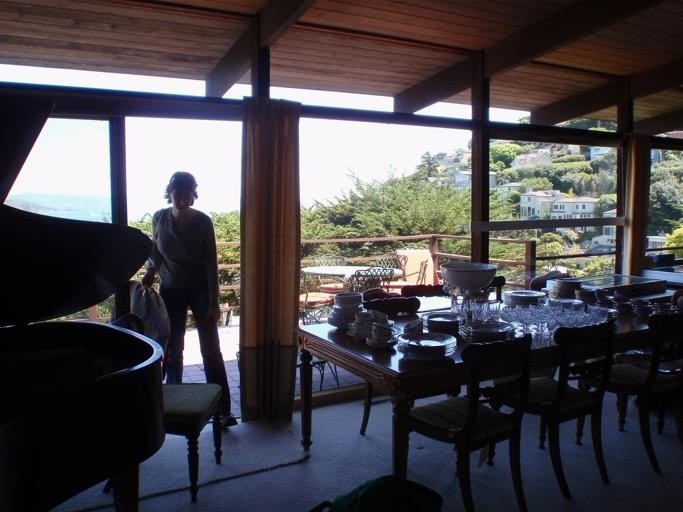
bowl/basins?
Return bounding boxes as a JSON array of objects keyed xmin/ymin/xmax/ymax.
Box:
[
  {"xmin": 440, "ymin": 261, "xmax": 498, "ymax": 295},
  {"xmin": 328, "ymin": 292, "xmax": 396, "ymax": 348},
  {"xmin": 572, "ymin": 288, "xmax": 681, "ymax": 320}
]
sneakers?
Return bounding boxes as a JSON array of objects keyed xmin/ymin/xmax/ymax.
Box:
[{"xmin": 220, "ymin": 415, "xmax": 238, "ymax": 427}]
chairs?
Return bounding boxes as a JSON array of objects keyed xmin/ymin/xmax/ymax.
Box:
[
  {"xmin": 359, "ymin": 271, "xmax": 682, "ymax": 510},
  {"xmin": 102, "ymin": 313, "xmax": 224, "ymax": 502}
]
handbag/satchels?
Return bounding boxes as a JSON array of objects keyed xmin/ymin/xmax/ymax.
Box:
[{"xmin": 148, "ymin": 240, "xmax": 161, "ymax": 272}]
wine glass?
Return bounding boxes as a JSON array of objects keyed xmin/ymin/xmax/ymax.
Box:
[{"xmin": 459, "ymin": 297, "xmax": 609, "ymax": 348}]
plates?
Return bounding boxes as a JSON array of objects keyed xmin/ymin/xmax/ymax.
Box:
[
  {"xmin": 506, "ymin": 277, "xmax": 582, "ymax": 308},
  {"xmin": 397, "ymin": 312, "xmax": 516, "ymax": 362}
]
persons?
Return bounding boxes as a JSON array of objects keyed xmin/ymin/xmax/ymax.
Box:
[{"xmin": 142, "ymin": 171, "xmax": 239, "ymax": 430}]
